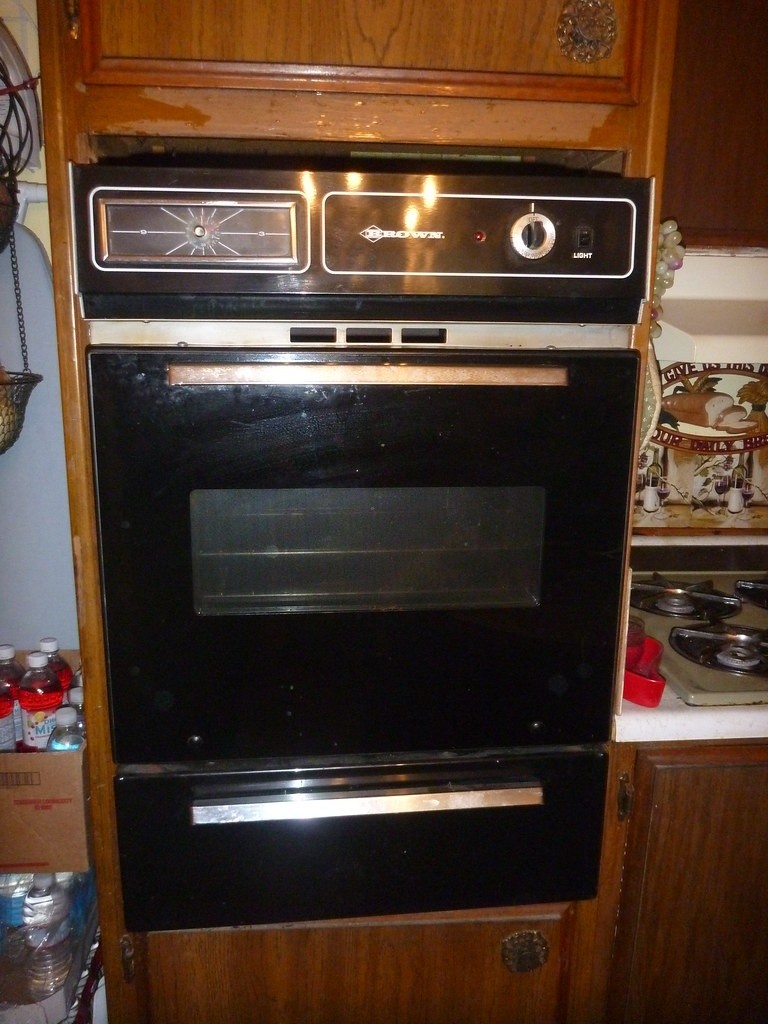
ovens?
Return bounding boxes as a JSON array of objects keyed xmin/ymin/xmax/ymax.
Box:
[{"xmin": 68, "ymin": 160, "xmax": 657, "ymax": 768}]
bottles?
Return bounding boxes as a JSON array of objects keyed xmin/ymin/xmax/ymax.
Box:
[
  {"xmin": 39, "ymin": 637, "xmax": 74, "ymax": 708},
  {"xmin": 66, "ymin": 686, "xmax": 84, "ymax": 734},
  {"xmin": 0, "ymin": 680, "xmax": 17, "ymax": 753},
  {"xmin": 72, "ymin": 660, "xmax": 84, "ymax": 687},
  {"xmin": 22, "ymin": 872, "xmax": 72, "ymax": 1002},
  {"xmin": 18, "ymin": 651, "xmax": 64, "ymax": 751},
  {"xmin": 727, "ymin": 452, "xmax": 748, "ymax": 513},
  {"xmin": 642, "ymin": 447, "xmax": 663, "ymax": 513},
  {"xmin": 0, "ymin": 644, "xmax": 27, "ymax": 753},
  {"xmin": 46, "ymin": 706, "xmax": 85, "ymax": 751}
]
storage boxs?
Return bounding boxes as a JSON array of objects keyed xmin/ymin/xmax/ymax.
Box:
[{"xmin": 0, "ymin": 648, "xmax": 91, "ymax": 874}]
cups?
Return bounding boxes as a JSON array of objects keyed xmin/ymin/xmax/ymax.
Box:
[
  {"xmin": 625, "ymin": 615, "xmax": 645, "ymax": 672},
  {"xmin": 727, "ymin": 486, "xmax": 745, "ymax": 514},
  {"xmin": 642, "ymin": 484, "xmax": 661, "ymax": 513}
]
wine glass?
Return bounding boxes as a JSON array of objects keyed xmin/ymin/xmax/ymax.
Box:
[
  {"xmin": 738, "ymin": 479, "xmax": 755, "ymax": 520},
  {"xmin": 653, "ymin": 477, "xmax": 671, "ymax": 519},
  {"xmin": 714, "ymin": 480, "xmax": 728, "ymax": 514},
  {"xmin": 633, "ymin": 474, "xmax": 647, "ymax": 521}
]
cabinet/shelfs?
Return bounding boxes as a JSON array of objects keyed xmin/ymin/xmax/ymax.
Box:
[{"xmin": 36, "ymin": 0, "xmax": 768, "ymax": 1024}]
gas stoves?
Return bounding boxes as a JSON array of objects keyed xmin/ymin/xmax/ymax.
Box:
[{"xmin": 631, "ymin": 570, "xmax": 768, "ymax": 705}]
